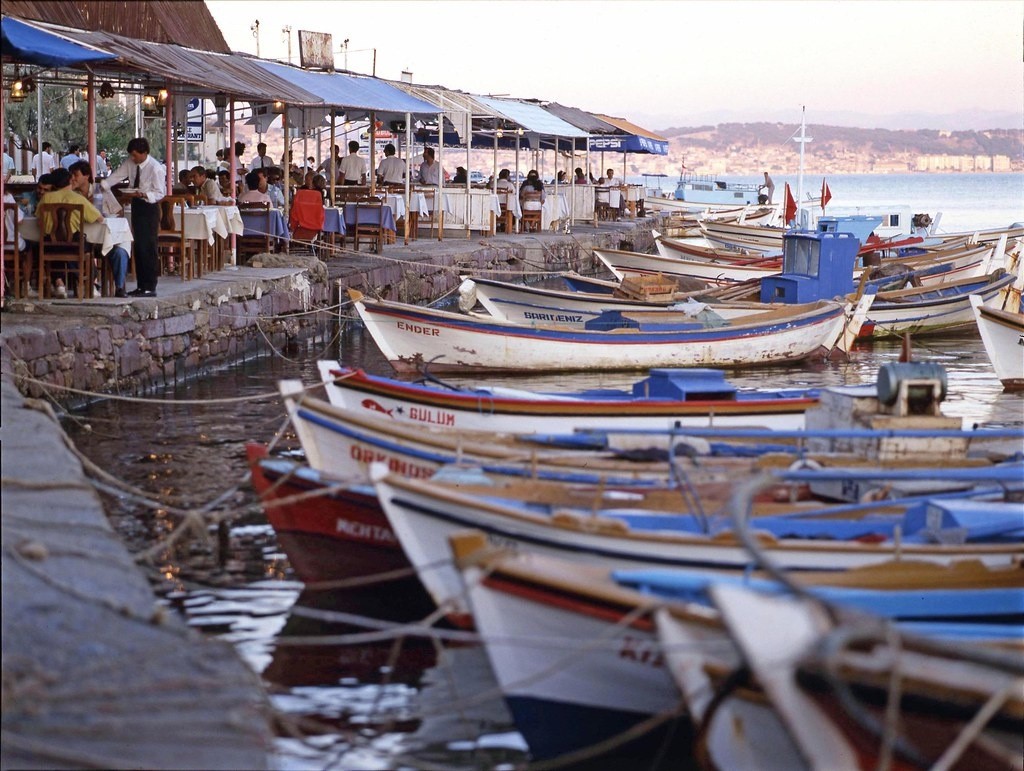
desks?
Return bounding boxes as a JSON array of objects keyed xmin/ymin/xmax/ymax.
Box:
[
  {"xmin": 621, "ymin": 187, "xmax": 646, "ymax": 217},
  {"xmin": 424, "ymin": 193, "xmax": 452, "ymax": 239},
  {"xmin": 500, "ymin": 193, "xmax": 523, "ymax": 234},
  {"xmin": 16, "ymin": 216, "xmax": 134, "ymax": 300},
  {"xmin": 124, "ymin": 206, "xmax": 228, "ymax": 282},
  {"xmin": 364, "ymin": 195, "xmax": 406, "ymax": 225},
  {"xmin": 540, "ymin": 194, "xmax": 571, "ymax": 234},
  {"xmin": 597, "ymin": 188, "xmax": 621, "ymax": 222},
  {"xmin": 241, "ymin": 208, "xmax": 290, "ymax": 246},
  {"xmin": 322, "ymin": 208, "xmax": 346, "ymax": 256},
  {"xmin": 195, "ymin": 205, "xmax": 244, "ymax": 273},
  {"xmin": 335, "ymin": 203, "xmax": 397, "ymax": 253},
  {"xmin": 400, "ymin": 193, "xmax": 429, "ymax": 241},
  {"xmin": 416, "ymin": 187, "xmax": 501, "ymax": 237}
]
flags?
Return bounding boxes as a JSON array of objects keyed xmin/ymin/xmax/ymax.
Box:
[
  {"xmin": 785, "ymin": 185, "xmax": 798, "ymax": 226},
  {"xmin": 821, "ymin": 181, "xmax": 832, "ymax": 209}
]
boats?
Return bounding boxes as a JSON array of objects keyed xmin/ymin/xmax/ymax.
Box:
[{"xmin": 244, "ymin": 104, "xmax": 1024, "ymax": 771}]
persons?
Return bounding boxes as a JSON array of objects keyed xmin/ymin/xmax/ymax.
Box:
[
  {"xmin": 105, "ymin": 137, "xmax": 166, "ymax": 297},
  {"xmin": 60, "ymin": 144, "xmax": 81, "ymax": 170},
  {"xmin": 216, "ymin": 141, "xmax": 284, "ymax": 260},
  {"xmin": 316, "ymin": 145, "xmax": 342, "ymax": 183},
  {"xmin": 85, "ymin": 144, "xmax": 111, "ymax": 176},
  {"xmin": 550, "ymin": 167, "xmax": 626, "ymax": 221},
  {"xmin": 418, "ymin": 147, "xmax": 444, "ymax": 185},
  {"xmin": 377, "ymin": 144, "xmax": 405, "ymax": 186},
  {"xmin": 453, "ymin": 167, "xmax": 467, "ymax": 183},
  {"xmin": 488, "ymin": 168, "xmax": 546, "ymax": 232},
  {"xmin": 32, "ymin": 142, "xmax": 54, "ymax": 182},
  {"xmin": 173, "ymin": 166, "xmax": 230, "ymax": 206},
  {"xmin": 338, "ymin": 140, "xmax": 367, "ymax": 185},
  {"xmin": 3, "ymin": 158, "xmax": 128, "ymax": 299},
  {"xmin": 3, "ymin": 144, "xmax": 16, "ymax": 182},
  {"xmin": 281, "ymin": 150, "xmax": 332, "ymax": 206},
  {"xmin": 760, "ymin": 172, "xmax": 775, "ymax": 205}
]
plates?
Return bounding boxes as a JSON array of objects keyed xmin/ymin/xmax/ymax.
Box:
[{"xmin": 117, "ymin": 188, "xmax": 139, "ymax": 193}]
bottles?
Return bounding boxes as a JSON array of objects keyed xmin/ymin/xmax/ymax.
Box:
[
  {"xmin": 194, "ymin": 188, "xmax": 201, "ymax": 206},
  {"xmin": 93, "ymin": 184, "xmax": 103, "ymax": 213}
]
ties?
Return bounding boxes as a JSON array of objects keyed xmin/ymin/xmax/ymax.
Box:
[
  {"xmin": 261, "ymin": 157, "xmax": 264, "ymax": 168},
  {"xmin": 134, "ymin": 166, "xmax": 140, "ymax": 189}
]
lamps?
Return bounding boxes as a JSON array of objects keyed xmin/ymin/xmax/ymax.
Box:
[{"xmin": 10, "ymin": 65, "xmax": 525, "ymax": 138}]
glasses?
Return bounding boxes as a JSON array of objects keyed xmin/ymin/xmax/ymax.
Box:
[{"xmin": 272, "ymin": 178, "xmax": 279, "ymax": 181}]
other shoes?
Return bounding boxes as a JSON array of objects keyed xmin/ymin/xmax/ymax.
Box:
[
  {"xmin": 617, "ymin": 217, "xmax": 621, "ymax": 222},
  {"xmin": 21, "ymin": 288, "xmax": 38, "ymax": 298},
  {"xmin": 528, "ymin": 224, "xmax": 536, "ymax": 233},
  {"xmin": 625, "ymin": 208, "xmax": 633, "ymax": 216},
  {"xmin": 500, "ymin": 222, "xmax": 505, "ymax": 232},
  {"xmin": 55, "ymin": 286, "xmax": 68, "ymax": 299},
  {"xmin": 93, "ymin": 286, "xmax": 101, "ymax": 297}
]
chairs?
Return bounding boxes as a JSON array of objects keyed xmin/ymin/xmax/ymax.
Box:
[{"xmin": 1, "ymin": 185, "xmax": 645, "ymax": 298}]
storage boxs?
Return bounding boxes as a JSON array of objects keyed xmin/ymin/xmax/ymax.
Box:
[{"xmin": 613, "ymin": 272, "xmax": 679, "ymax": 302}]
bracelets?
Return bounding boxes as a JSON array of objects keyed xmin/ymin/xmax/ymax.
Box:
[{"xmin": 142, "ymin": 193, "xmax": 143, "ymax": 198}]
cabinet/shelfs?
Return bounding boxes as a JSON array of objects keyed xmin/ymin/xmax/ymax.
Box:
[{"xmin": 806, "ymin": 387, "xmax": 968, "ymax": 504}]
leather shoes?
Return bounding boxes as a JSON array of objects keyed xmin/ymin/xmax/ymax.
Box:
[
  {"xmin": 133, "ymin": 290, "xmax": 156, "ymax": 297},
  {"xmin": 116, "ymin": 289, "xmax": 128, "ymax": 298},
  {"xmin": 128, "ymin": 288, "xmax": 141, "ymax": 295}
]
